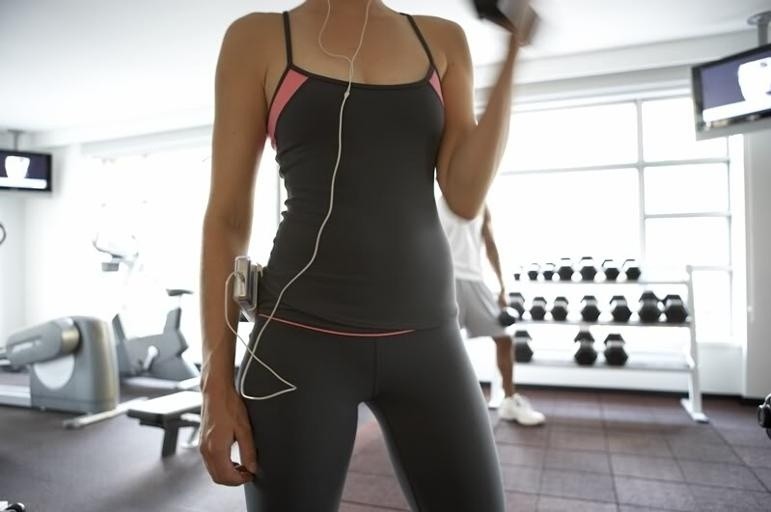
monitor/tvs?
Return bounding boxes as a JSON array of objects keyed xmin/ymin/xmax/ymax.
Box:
[
  {"xmin": 0, "ymin": 148, "xmax": 51, "ymax": 192},
  {"xmin": 689, "ymin": 42, "xmax": 770, "ymax": 140}
]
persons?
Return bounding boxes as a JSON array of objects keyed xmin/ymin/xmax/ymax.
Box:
[
  {"xmin": 428, "ymin": 173, "xmax": 547, "ymax": 425},
  {"xmin": 199, "ymin": 0, "xmax": 535, "ymax": 510}
]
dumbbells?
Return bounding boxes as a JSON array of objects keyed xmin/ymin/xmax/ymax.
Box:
[
  {"xmin": 510, "ymin": 330, "xmax": 533, "ymax": 364},
  {"xmin": 572, "ymin": 330, "xmax": 596, "ymax": 369},
  {"xmin": 496, "ymin": 254, "xmax": 640, "ymax": 281},
  {"xmin": 601, "ymin": 333, "xmax": 629, "ymax": 369},
  {"xmin": 502, "ymin": 292, "xmax": 690, "ymax": 326}
]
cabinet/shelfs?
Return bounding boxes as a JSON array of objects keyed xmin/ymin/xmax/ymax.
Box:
[{"xmin": 487, "ymin": 264, "xmax": 709, "ymax": 424}]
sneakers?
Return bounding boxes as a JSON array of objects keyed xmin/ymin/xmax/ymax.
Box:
[{"xmin": 499, "ymin": 392, "xmax": 546, "ymax": 427}]
